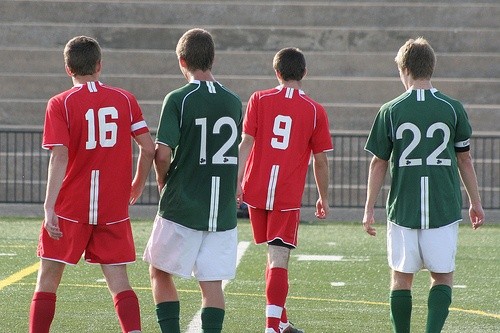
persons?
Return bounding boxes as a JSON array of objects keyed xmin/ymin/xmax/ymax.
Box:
[
  {"xmin": 30, "ymin": 35, "xmax": 156, "ymax": 332},
  {"xmin": 362, "ymin": 35, "xmax": 485, "ymax": 333},
  {"xmin": 142, "ymin": 29, "xmax": 243, "ymax": 333},
  {"xmin": 236, "ymin": 46, "xmax": 332, "ymax": 333}
]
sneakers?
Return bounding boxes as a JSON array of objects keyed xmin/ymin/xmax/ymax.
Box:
[{"xmin": 279, "ymin": 321, "xmax": 304, "ymax": 333}]
